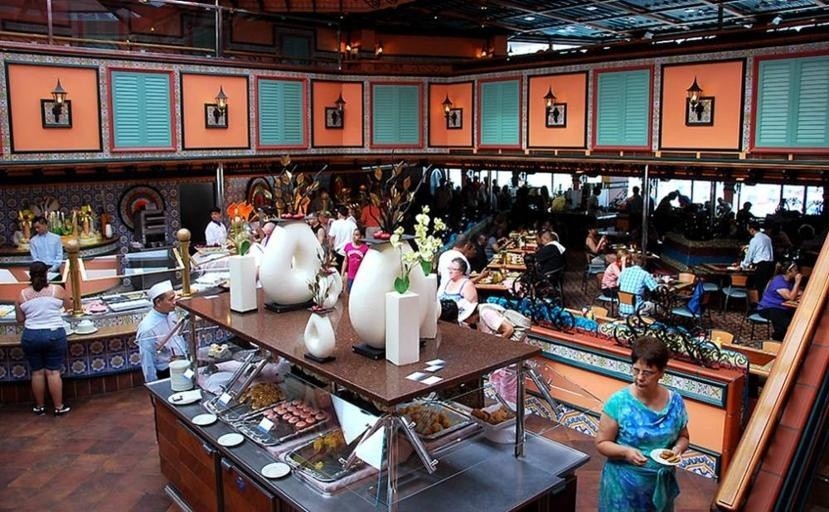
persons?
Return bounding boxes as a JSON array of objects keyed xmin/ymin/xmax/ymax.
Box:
[
  {"xmin": 594, "ymin": 336, "xmax": 690, "ymax": 512},
  {"xmin": 204, "ymin": 207, "xmax": 276, "ymax": 256},
  {"xmin": 136, "ymin": 278, "xmax": 188, "ymax": 384},
  {"xmin": 15, "ymin": 260, "xmax": 70, "ymax": 415},
  {"xmin": 306, "ymin": 198, "xmax": 381, "ymax": 289},
  {"xmin": 28, "ymin": 215, "xmax": 66, "ymax": 290}
]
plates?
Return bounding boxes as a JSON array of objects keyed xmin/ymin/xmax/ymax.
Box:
[
  {"xmin": 74, "ymin": 327, "xmax": 98, "ymax": 334},
  {"xmin": 84, "ymin": 308, "xmax": 109, "ymax": 316},
  {"xmin": 650, "ymin": 448, "xmax": 683, "ymax": 466},
  {"xmin": 166, "ymin": 360, "xmax": 195, "ymax": 391},
  {"xmin": 64, "ymin": 331, "xmax": 74, "ymax": 336},
  {"xmin": 167, "ymin": 392, "xmax": 196, "ymax": 405},
  {"xmin": 191, "ymin": 413, "xmax": 218, "ymax": 426},
  {"xmin": 474, "ymin": 398, "xmax": 532, "ymax": 430},
  {"xmin": 260, "ymin": 462, "xmax": 291, "ymax": 479},
  {"xmin": 217, "ymin": 432, "xmax": 245, "ymax": 446}
]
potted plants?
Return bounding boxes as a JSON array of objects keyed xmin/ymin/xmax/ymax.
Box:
[
  {"xmin": 347, "ymin": 146, "xmax": 430, "ymax": 348},
  {"xmin": 259, "ymin": 154, "xmax": 329, "ymax": 305}
]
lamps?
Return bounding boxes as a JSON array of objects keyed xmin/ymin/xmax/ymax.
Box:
[
  {"xmin": 441, "ymin": 93, "xmax": 457, "ymax": 126},
  {"xmin": 213, "ymin": 84, "xmax": 228, "ymax": 124},
  {"xmin": 542, "ymin": 85, "xmax": 560, "ymax": 123},
  {"xmin": 52, "ymin": 79, "xmax": 68, "ymax": 123},
  {"xmin": 687, "ymin": 75, "xmax": 704, "ymax": 119},
  {"xmin": 332, "ymin": 91, "xmax": 346, "ymax": 125}
]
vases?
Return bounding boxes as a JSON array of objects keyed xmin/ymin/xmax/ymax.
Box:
[
  {"xmin": 385, "ymin": 291, "xmax": 420, "ymax": 366},
  {"xmin": 302, "ymin": 313, "xmax": 336, "ymax": 358},
  {"xmin": 228, "ymin": 254, "xmax": 259, "ymax": 313}
]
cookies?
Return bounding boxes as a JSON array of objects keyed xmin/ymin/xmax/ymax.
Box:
[{"xmin": 660, "ymin": 450, "xmax": 681, "ymax": 464}]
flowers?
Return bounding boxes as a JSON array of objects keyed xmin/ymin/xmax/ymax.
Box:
[
  {"xmin": 389, "ymin": 206, "xmax": 447, "ymax": 293},
  {"xmin": 225, "ymin": 200, "xmax": 254, "ymax": 252},
  {"xmin": 308, "ymin": 240, "xmax": 340, "ymax": 314}
]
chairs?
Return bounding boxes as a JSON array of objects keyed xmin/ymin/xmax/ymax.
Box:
[
  {"xmin": 471, "ymin": 234, "xmax": 488, "ymax": 265},
  {"xmin": 582, "ymin": 238, "xmax": 820, "ymax": 347},
  {"xmin": 537, "ymin": 268, "xmax": 564, "ymax": 305}
]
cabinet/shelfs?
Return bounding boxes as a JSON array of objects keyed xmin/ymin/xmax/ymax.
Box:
[
  {"xmin": 1, "ymin": 247, "xmax": 234, "ymax": 410},
  {"xmin": 144, "ymin": 288, "xmax": 591, "ymax": 511}
]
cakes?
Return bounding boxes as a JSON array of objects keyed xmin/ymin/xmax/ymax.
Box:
[
  {"xmin": 263, "ymin": 398, "xmax": 326, "ymax": 432},
  {"xmin": 207, "ymin": 343, "xmax": 230, "ymax": 358}
]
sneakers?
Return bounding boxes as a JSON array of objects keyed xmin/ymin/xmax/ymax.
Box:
[
  {"xmin": 31, "ymin": 405, "xmax": 47, "ymax": 416},
  {"xmin": 54, "ymin": 404, "xmax": 72, "ymax": 416}
]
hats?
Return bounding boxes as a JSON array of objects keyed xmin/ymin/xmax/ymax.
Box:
[
  {"xmin": 148, "ymin": 279, "xmax": 173, "ymax": 300},
  {"xmin": 456, "ymin": 298, "xmax": 479, "ymax": 323}
]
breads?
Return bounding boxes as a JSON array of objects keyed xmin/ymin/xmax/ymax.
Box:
[
  {"xmin": 469, "ymin": 405, "xmax": 515, "ymax": 425},
  {"xmin": 237, "ymin": 382, "xmax": 282, "ymax": 410},
  {"xmin": 314, "ymin": 430, "xmax": 347, "ymax": 450},
  {"xmin": 398, "ymin": 401, "xmax": 451, "ymax": 436}
]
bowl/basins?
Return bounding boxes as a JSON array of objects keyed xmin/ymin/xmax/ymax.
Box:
[
  {"xmin": 483, "ymin": 424, "xmax": 526, "ymax": 443},
  {"xmin": 205, "ymin": 371, "xmax": 234, "ymax": 393}
]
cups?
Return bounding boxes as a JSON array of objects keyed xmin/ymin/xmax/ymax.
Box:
[
  {"xmin": 500, "ymin": 266, "xmax": 506, "ymax": 280},
  {"xmin": 77, "ymin": 322, "xmax": 94, "ymax": 332},
  {"xmin": 502, "ymin": 253, "xmax": 517, "ymax": 265},
  {"xmin": 493, "ymin": 271, "xmax": 498, "ymax": 284},
  {"xmin": 63, "ymin": 323, "xmax": 70, "ymax": 332}
]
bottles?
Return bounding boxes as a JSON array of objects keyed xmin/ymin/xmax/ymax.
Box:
[
  {"xmin": 18, "ymin": 211, "xmax": 95, "ymax": 238},
  {"xmin": 105, "ymin": 219, "xmax": 113, "ymax": 240},
  {"xmin": 229, "ymin": 206, "xmax": 253, "ymax": 235}
]
